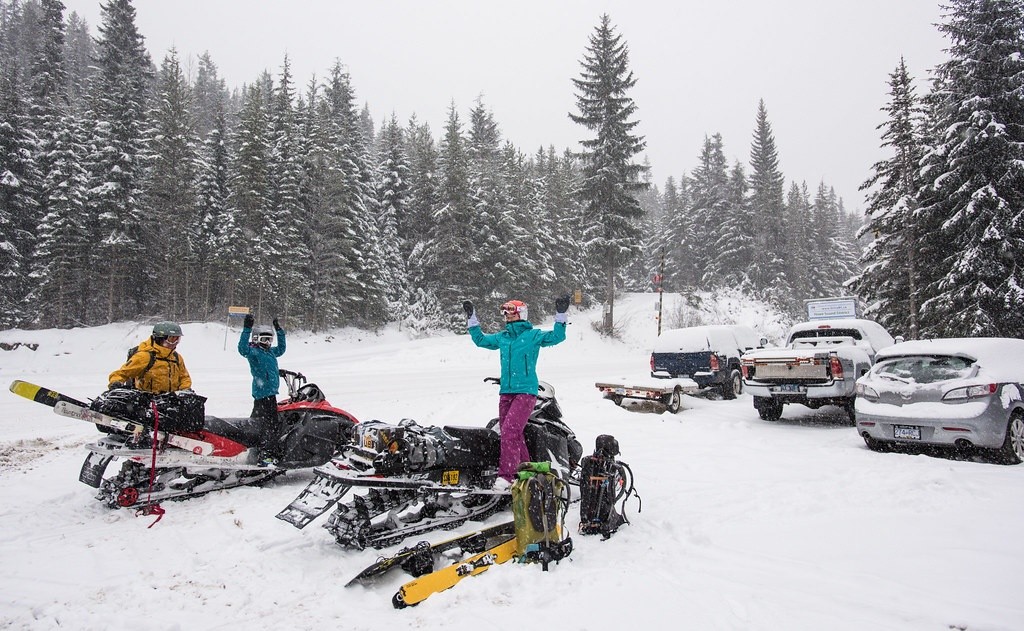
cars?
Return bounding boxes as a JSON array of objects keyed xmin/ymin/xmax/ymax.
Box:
[{"xmin": 855, "ymin": 352, "xmax": 1024, "ymax": 465}]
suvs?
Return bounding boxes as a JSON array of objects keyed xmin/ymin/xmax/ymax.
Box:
[{"xmin": 650, "ymin": 325, "xmax": 772, "ymax": 400}]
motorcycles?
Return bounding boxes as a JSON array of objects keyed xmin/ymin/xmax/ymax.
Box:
[
  {"xmin": 275, "ymin": 378, "xmax": 583, "ymax": 551},
  {"xmin": 80, "ymin": 369, "xmax": 359, "ymax": 512}
]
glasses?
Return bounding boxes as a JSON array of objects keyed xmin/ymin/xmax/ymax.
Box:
[{"xmin": 255, "ymin": 336, "xmax": 274, "ymax": 344}]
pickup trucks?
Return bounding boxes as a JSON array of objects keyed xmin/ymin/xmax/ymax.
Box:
[{"xmin": 741, "ymin": 319, "xmax": 905, "ymax": 421}]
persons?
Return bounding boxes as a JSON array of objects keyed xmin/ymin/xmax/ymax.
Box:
[
  {"xmin": 462, "ymin": 295, "xmax": 570, "ymax": 490},
  {"xmin": 108, "ymin": 321, "xmax": 191, "ymax": 394},
  {"xmin": 239, "ymin": 313, "xmax": 286, "ymax": 462}
]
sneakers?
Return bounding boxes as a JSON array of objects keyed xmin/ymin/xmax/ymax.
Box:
[{"xmin": 493, "ymin": 478, "xmax": 512, "ymax": 490}]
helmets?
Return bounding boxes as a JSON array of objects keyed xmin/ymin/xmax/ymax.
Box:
[
  {"xmin": 500, "ymin": 299, "xmax": 528, "ymax": 321},
  {"xmin": 252, "ymin": 325, "xmax": 274, "ymax": 347},
  {"xmin": 152, "ymin": 321, "xmax": 185, "ymax": 341}
]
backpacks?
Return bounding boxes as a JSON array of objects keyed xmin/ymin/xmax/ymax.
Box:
[
  {"xmin": 125, "ymin": 346, "xmax": 179, "ymax": 388},
  {"xmin": 580, "ymin": 450, "xmax": 631, "ymax": 537},
  {"xmin": 512, "ymin": 463, "xmax": 569, "ymax": 560}
]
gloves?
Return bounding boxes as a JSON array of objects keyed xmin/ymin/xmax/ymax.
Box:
[
  {"xmin": 555, "ymin": 294, "xmax": 570, "ymax": 323},
  {"xmin": 273, "ymin": 317, "xmax": 279, "ymax": 329},
  {"xmin": 461, "ymin": 300, "xmax": 479, "ymax": 326},
  {"xmin": 244, "ymin": 314, "xmax": 254, "ymax": 328},
  {"xmin": 110, "ymin": 381, "xmax": 121, "ymax": 390}
]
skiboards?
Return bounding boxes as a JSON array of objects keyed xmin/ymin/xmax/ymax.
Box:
[
  {"xmin": 344, "ymin": 521, "xmax": 518, "ymax": 610},
  {"xmin": 8, "ymin": 378, "xmax": 215, "ymax": 457}
]
